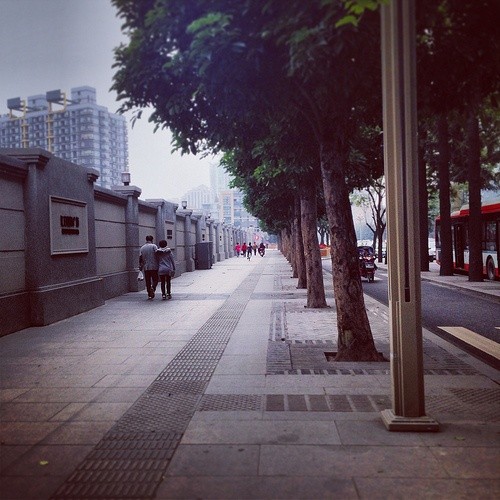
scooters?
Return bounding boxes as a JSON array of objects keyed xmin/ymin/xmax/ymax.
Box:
[
  {"xmin": 259, "ymin": 247, "xmax": 265, "ymax": 257},
  {"xmin": 357, "ymin": 245, "xmax": 377, "ymax": 283}
]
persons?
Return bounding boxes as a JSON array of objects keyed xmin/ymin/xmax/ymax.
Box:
[
  {"xmin": 242, "ymin": 242, "xmax": 247, "ymax": 257},
  {"xmin": 157, "ymin": 240, "xmax": 176, "ymax": 300},
  {"xmin": 235, "ymin": 242, "xmax": 241, "ymax": 257},
  {"xmin": 139, "ymin": 235, "xmax": 173, "ymax": 300},
  {"xmin": 246, "ymin": 242, "xmax": 253, "ymax": 259},
  {"xmin": 252, "ymin": 242, "xmax": 258, "ymax": 256},
  {"xmin": 358, "ymin": 226, "xmax": 500, "ymax": 267},
  {"xmin": 258, "ymin": 242, "xmax": 265, "ymax": 254}
]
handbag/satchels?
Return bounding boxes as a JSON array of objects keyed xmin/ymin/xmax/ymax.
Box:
[
  {"xmin": 169, "ymin": 269, "xmax": 174, "ymax": 277},
  {"xmin": 137, "ymin": 271, "xmax": 144, "ymax": 281}
]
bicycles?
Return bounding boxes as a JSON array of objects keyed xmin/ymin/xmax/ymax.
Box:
[{"xmin": 248, "ymin": 252, "xmax": 251, "ymax": 262}]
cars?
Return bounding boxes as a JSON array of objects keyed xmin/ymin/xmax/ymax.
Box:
[
  {"xmin": 357, "ymin": 240, "xmax": 386, "ymax": 258},
  {"xmin": 428, "ymin": 238, "xmax": 436, "ymax": 262}
]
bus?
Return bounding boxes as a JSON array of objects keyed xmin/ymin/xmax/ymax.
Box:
[{"xmin": 434, "ymin": 198, "xmax": 500, "ymax": 280}]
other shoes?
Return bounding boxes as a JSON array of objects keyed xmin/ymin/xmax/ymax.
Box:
[
  {"xmin": 148, "ymin": 287, "xmax": 155, "ymax": 299},
  {"xmin": 163, "ymin": 294, "xmax": 166, "ymax": 300},
  {"xmin": 167, "ymin": 294, "xmax": 171, "ymax": 299}
]
